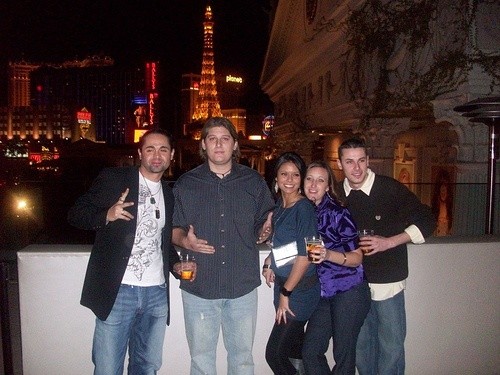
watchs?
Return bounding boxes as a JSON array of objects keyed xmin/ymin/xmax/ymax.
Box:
[{"xmin": 263, "ymin": 265, "xmax": 272, "ymax": 268}]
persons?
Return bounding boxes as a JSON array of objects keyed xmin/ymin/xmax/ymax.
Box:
[
  {"xmin": 300, "ymin": 161, "xmax": 371, "ymax": 375},
  {"xmin": 330, "ymin": 138, "xmax": 436, "ymax": 375},
  {"xmin": 258, "ymin": 153, "xmax": 320, "ymax": 375},
  {"xmin": 171, "ymin": 116, "xmax": 274, "ymax": 375},
  {"xmin": 68, "ymin": 126, "xmax": 198, "ymax": 375}
]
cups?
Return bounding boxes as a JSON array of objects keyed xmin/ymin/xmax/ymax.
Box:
[
  {"xmin": 356, "ymin": 229, "xmax": 374, "ymax": 254},
  {"xmin": 303, "ymin": 236, "xmax": 323, "ymax": 263},
  {"xmin": 179, "ymin": 251, "xmax": 198, "ymax": 279}
]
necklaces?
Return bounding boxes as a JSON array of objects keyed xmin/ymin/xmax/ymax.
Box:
[{"xmin": 143, "ymin": 174, "xmax": 164, "ymax": 220}]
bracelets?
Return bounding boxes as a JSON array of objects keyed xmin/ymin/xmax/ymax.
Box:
[
  {"xmin": 342, "ymin": 252, "xmax": 347, "ymax": 265},
  {"xmin": 280, "ymin": 286, "xmax": 292, "ymax": 297}
]
